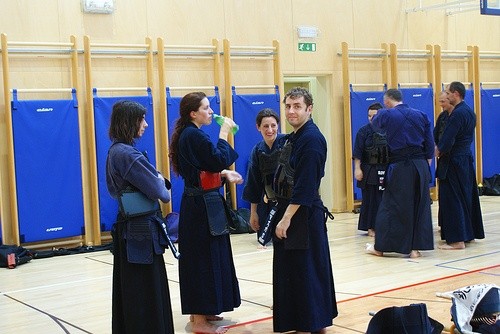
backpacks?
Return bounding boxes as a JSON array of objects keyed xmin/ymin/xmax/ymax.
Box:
[
  {"xmin": 165, "ymin": 212, "xmax": 181, "ymax": 244},
  {"xmin": 228, "ymin": 207, "xmax": 254, "ymax": 235},
  {"xmin": 0, "ymin": 245, "xmax": 33, "ymax": 269}
]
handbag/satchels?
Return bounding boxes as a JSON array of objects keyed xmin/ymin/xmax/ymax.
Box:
[
  {"xmin": 200, "ymin": 170, "xmax": 222, "ymax": 192},
  {"xmin": 484, "ymin": 173, "xmax": 500, "ymax": 195},
  {"xmin": 121, "ymin": 189, "xmax": 160, "ymax": 217}
]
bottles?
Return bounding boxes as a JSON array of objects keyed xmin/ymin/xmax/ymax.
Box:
[{"xmin": 213, "ymin": 113, "xmax": 239, "ymax": 135}]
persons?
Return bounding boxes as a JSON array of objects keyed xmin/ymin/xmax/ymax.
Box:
[
  {"xmin": 271, "ymin": 87, "xmax": 339, "ymax": 334},
  {"xmin": 105, "ymin": 99, "xmax": 177, "ymax": 334},
  {"xmin": 438, "ymin": 81, "xmax": 485, "ymax": 250},
  {"xmin": 241, "ymin": 107, "xmax": 291, "ymax": 232},
  {"xmin": 365, "ymin": 89, "xmax": 436, "ymax": 258},
  {"xmin": 433, "ymin": 92, "xmax": 455, "ymax": 145},
  {"xmin": 352, "ymin": 103, "xmax": 390, "ymax": 236},
  {"xmin": 168, "ymin": 91, "xmax": 244, "ymax": 334}
]
